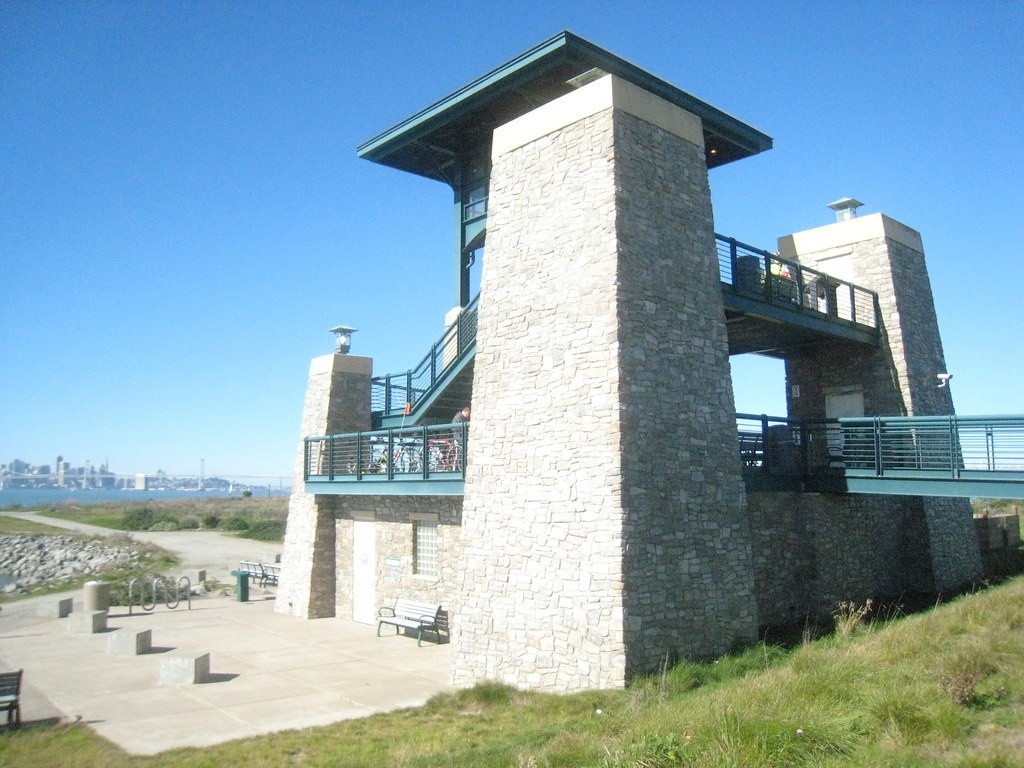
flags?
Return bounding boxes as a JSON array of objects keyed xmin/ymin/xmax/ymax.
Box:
[{"xmin": 403, "ymin": 402, "xmax": 411, "ymax": 415}]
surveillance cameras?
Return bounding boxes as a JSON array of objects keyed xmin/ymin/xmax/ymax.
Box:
[{"xmin": 937, "ymin": 374, "xmax": 953, "ymax": 379}]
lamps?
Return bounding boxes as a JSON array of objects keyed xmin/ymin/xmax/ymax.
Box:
[{"xmin": 329, "ymin": 325, "xmax": 359, "ymax": 355}]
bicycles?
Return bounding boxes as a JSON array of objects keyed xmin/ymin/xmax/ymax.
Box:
[{"xmin": 362, "ymin": 431, "xmax": 463, "ymax": 473}]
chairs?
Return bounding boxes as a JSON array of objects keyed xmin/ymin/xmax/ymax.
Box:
[{"xmin": 826, "ymin": 434, "xmax": 871, "ymax": 467}]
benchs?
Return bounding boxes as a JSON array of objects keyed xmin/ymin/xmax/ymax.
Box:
[
  {"xmin": 738, "ymin": 432, "xmax": 763, "ymax": 467},
  {"xmin": 762, "ymin": 273, "xmax": 807, "ymax": 304},
  {"xmin": 375, "ymin": 597, "xmax": 442, "ymax": 647},
  {"xmin": 264, "ymin": 564, "xmax": 281, "ymax": 587},
  {"xmin": 240, "ymin": 561, "xmax": 274, "ymax": 588},
  {"xmin": 0, "ymin": 668, "xmax": 24, "ymax": 728}
]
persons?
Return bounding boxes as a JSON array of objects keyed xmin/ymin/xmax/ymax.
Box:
[
  {"xmin": 760, "ymin": 251, "xmax": 782, "ymax": 284},
  {"xmin": 451, "ymin": 406, "xmax": 471, "ymax": 452}
]
